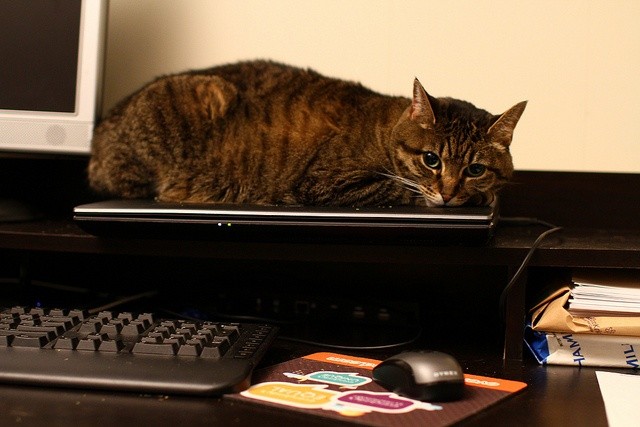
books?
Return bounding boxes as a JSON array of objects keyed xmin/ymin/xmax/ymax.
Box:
[{"xmin": 567, "ymin": 276, "xmax": 640, "ymax": 318}]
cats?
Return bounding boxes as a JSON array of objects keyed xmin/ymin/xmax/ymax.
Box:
[{"xmin": 87, "ymin": 56, "xmax": 530, "ymax": 212}]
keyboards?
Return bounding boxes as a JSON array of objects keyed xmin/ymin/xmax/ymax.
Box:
[{"xmin": 2, "ymin": 303, "xmax": 280, "ymax": 394}]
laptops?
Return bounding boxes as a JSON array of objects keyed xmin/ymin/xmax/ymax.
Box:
[{"xmin": 70, "ymin": 196, "xmax": 498, "ymax": 234}]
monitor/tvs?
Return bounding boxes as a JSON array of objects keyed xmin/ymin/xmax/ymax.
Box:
[{"xmin": 0, "ymin": 0, "xmax": 110, "ymax": 223}]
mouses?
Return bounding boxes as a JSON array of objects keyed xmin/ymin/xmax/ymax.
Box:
[{"xmin": 371, "ymin": 348, "xmax": 466, "ymax": 403}]
